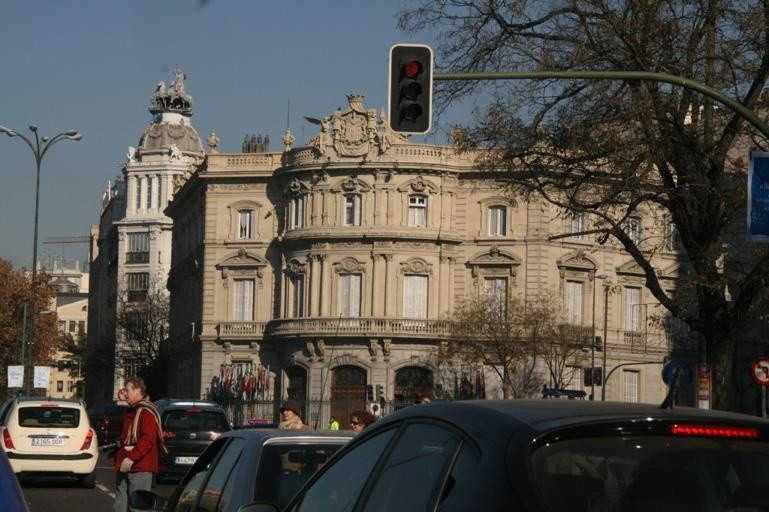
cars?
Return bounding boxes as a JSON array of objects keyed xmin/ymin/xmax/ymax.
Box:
[
  {"xmin": 128, "ymin": 423, "xmax": 368, "ymax": 511},
  {"xmin": 1, "ymin": 396, "xmax": 102, "ymax": 490}
]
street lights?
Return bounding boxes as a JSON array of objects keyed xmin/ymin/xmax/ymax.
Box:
[
  {"xmin": 592, "ymin": 274, "xmax": 608, "ymax": 398},
  {"xmin": 1, "ymin": 121, "xmax": 84, "ymax": 390}
]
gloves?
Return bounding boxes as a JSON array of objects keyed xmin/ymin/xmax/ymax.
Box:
[{"xmin": 119, "ymin": 458, "xmax": 133, "ymax": 473}]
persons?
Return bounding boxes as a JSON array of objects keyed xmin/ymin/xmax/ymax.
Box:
[
  {"xmin": 374, "ymin": 112, "xmax": 391, "ymax": 153},
  {"xmin": 242, "ymin": 133, "xmax": 269, "ymax": 153},
  {"xmin": 328, "ymin": 415, "xmax": 339, "ymax": 430},
  {"xmin": 111, "ymin": 376, "xmax": 168, "ymax": 512},
  {"xmin": 417, "ymin": 395, "xmax": 433, "ymax": 404},
  {"xmin": 315, "ymin": 117, "xmax": 331, "ymax": 153},
  {"xmin": 276, "ymin": 398, "xmax": 310, "ymax": 473},
  {"xmin": 172, "ymin": 67, "xmax": 186, "ymax": 98},
  {"xmin": 351, "ymin": 410, "xmax": 376, "ymax": 432}
]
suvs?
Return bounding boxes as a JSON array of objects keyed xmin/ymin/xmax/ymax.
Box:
[
  {"xmin": 289, "ymin": 389, "xmax": 768, "ymax": 510},
  {"xmin": 144, "ymin": 396, "xmax": 232, "ymax": 482}
]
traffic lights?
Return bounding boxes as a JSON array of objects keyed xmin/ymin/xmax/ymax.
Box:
[{"xmin": 387, "ymin": 43, "xmax": 432, "ymax": 137}]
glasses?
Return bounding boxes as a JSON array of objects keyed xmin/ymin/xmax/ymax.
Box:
[{"xmin": 352, "ymin": 422, "xmax": 359, "ymax": 425}]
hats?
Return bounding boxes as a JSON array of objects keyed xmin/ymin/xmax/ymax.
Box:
[{"xmin": 280, "ymin": 400, "xmax": 300, "ymax": 414}]
